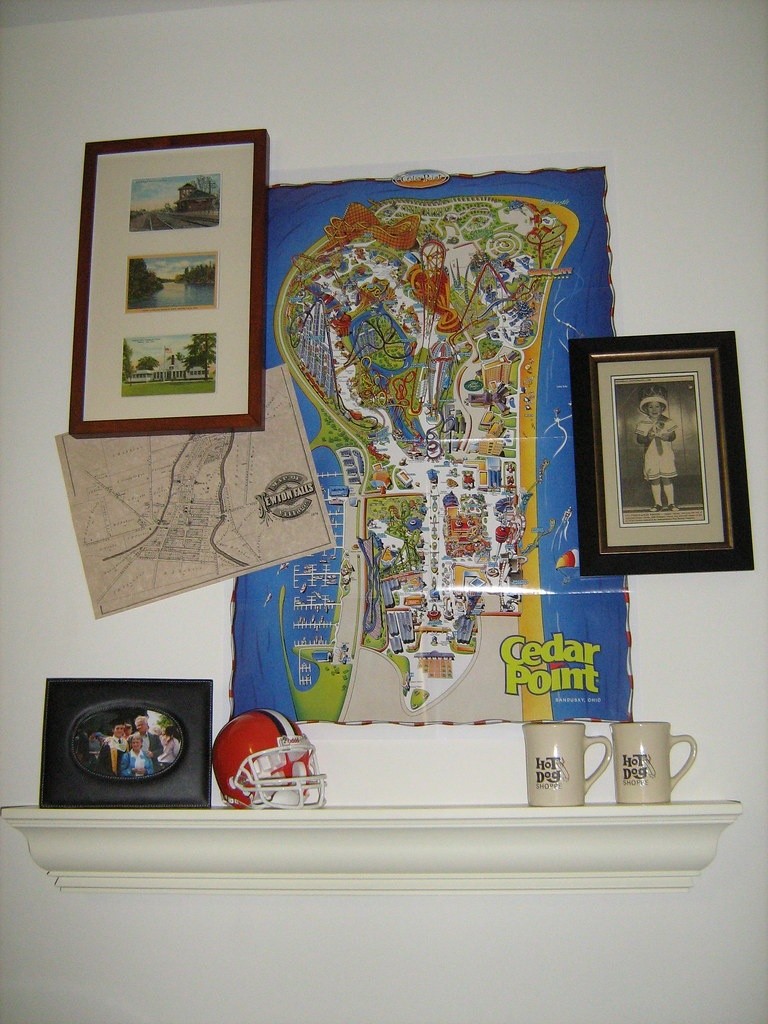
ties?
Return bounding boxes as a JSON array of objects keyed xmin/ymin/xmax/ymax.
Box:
[
  {"xmin": 118, "ymin": 739, "xmax": 121, "ymax": 743},
  {"xmin": 654, "ymin": 425, "xmax": 663, "ymax": 456}
]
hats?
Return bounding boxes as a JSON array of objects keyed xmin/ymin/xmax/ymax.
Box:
[
  {"xmin": 638, "ymin": 384, "xmax": 668, "ymax": 414},
  {"xmin": 108, "ymin": 717, "xmax": 125, "ymax": 728}
]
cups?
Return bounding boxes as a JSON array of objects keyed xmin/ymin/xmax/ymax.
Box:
[
  {"xmin": 523, "ymin": 724, "xmax": 612, "ymax": 806},
  {"xmin": 612, "ymin": 722, "xmax": 697, "ymax": 804}
]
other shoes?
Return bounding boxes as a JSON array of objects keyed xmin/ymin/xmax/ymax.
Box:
[
  {"xmin": 649, "ymin": 504, "xmax": 663, "ymax": 512},
  {"xmin": 668, "ymin": 504, "xmax": 680, "ymax": 511}
]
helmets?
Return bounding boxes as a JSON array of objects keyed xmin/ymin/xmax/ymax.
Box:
[{"xmin": 211, "ymin": 709, "xmax": 315, "ymax": 809}]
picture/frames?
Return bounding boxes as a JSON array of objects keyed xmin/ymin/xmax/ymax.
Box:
[
  {"xmin": 567, "ymin": 330, "xmax": 755, "ymax": 578},
  {"xmin": 69, "ymin": 128, "xmax": 271, "ymax": 439},
  {"xmin": 39, "ymin": 678, "xmax": 213, "ymax": 809}
]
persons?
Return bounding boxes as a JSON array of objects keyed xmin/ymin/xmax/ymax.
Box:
[
  {"xmin": 635, "ymin": 385, "xmax": 681, "ymax": 515},
  {"xmin": 73, "ymin": 716, "xmax": 181, "ymax": 779}
]
